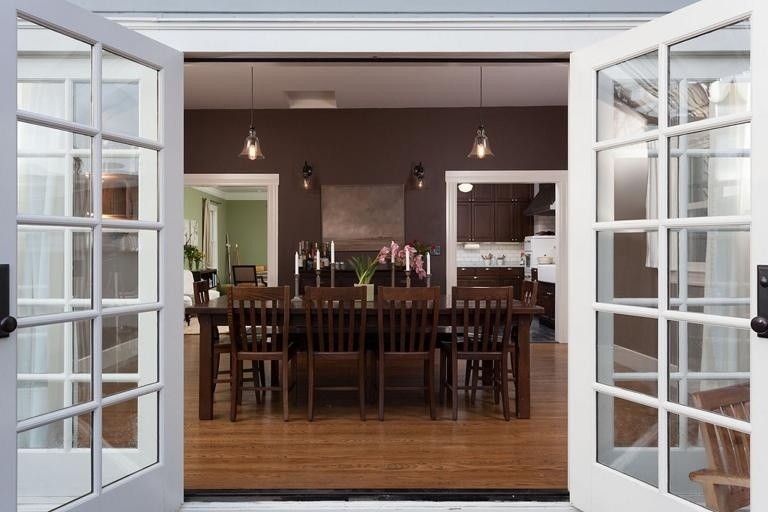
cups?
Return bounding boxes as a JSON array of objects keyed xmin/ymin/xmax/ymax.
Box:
[
  {"xmin": 483, "ymin": 259, "xmax": 492, "ymax": 266},
  {"xmin": 497, "ymin": 259, "xmax": 504, "ymax": 266}
]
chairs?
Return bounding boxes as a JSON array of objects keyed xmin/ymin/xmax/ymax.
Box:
[
  {"xmin": 688, "ymin": 380, "xmax": 749, "ymax": 512},
  {"xmin": 233, "ymin": 265, "xmax": 267, "ymax": 287},
  {"xmin": 192, "ymin": 280, "xmax": 305, "ymax": 421},
  {"xmin": 466, "ymin": 277, "xmax": 543, "ymax": 404},
  {"xmin": 184, "ymin": 270, "xmax": 220, "ymax": 308}
]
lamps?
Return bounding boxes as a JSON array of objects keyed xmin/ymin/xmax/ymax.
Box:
[
  {"xmin": 466, "ymin": 61, "xmax": 494, "ymax": 159},
  {"xmin": 411, "ymin": 161, "xmax": 425, "ymax": 190},
  {"xmin": 239, "ymin": 67, "xmax": 266, "ymax": 160},
  {"xmin": 300, "ymin": 161, "xmax": 313, "ymax": 190}
]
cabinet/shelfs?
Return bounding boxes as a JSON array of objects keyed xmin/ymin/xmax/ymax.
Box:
[
  {"xmin": 456, "ymin": 184, "xmax": 536, "ymax": 242},
  {"xmin": 457, "ymin": 266, "xmax": 523, "ymax": 299}
]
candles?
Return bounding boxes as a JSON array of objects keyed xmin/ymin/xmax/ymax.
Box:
[
  {"xmin": 426, "ymin": 252, "xmax": 431, "ymax": 275},
  {"xmin": 294, "ymin": 250, "xmax": 300, "ymax": 275},
  {"xmin": 390, "ymin": 249, "xmax": 396, "ymax": 263},
  {"xmin": 330, "ymin": 240, "xmax": 337, "ymax": 264},
  {"xmin": 316, "ymin": 250, "xmax": 322, "ymax": 269},
  {"xmin": 405, "ymin": 248, "xmax": 411, "ymax": 271}
]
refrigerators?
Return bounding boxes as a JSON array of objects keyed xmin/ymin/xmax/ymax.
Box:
[{"xmin": 524, "ymin": 235, "xmax": 556, "ymax": 297}]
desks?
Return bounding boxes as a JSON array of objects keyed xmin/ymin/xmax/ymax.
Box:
[{"xmin": 185, "ymin": 279, "xmax": 544, "ymax": 419}]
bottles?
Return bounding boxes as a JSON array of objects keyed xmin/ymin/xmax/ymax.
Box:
[{"xmin": 298, "ymin": 239, "xmax": 345, "ymax": 273}]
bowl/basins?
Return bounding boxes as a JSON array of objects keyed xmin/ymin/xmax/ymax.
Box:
[{"xmin": 536, "ymin": 256, "xmax": 554, "ymax": 264}]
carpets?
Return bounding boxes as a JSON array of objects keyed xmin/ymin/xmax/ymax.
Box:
[
  {"xmin": 183, "ymin": 317, "xmax": 244, "ymax": 334},
  {"xmin": 456, "ymin": 318, "xmax": 554, "ymax": 344}
]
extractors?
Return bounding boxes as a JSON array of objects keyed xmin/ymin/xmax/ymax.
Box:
[{"xmin": 522, "ymin": 183, "xmax": 556, "ymax": 217}]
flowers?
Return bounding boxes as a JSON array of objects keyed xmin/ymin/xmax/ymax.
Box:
[{"xmin": 359, "ymin": 239, "xmax": 437, "ymax": 284}]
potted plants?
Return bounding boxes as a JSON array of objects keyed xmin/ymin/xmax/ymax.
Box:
[
  {"xmin": 347, "ymin": 256, "xmax": 380, "ymax": 301},
  {"xmin": 184, "ymin": 244, "xmax": 212, "ymax": 282}
]
